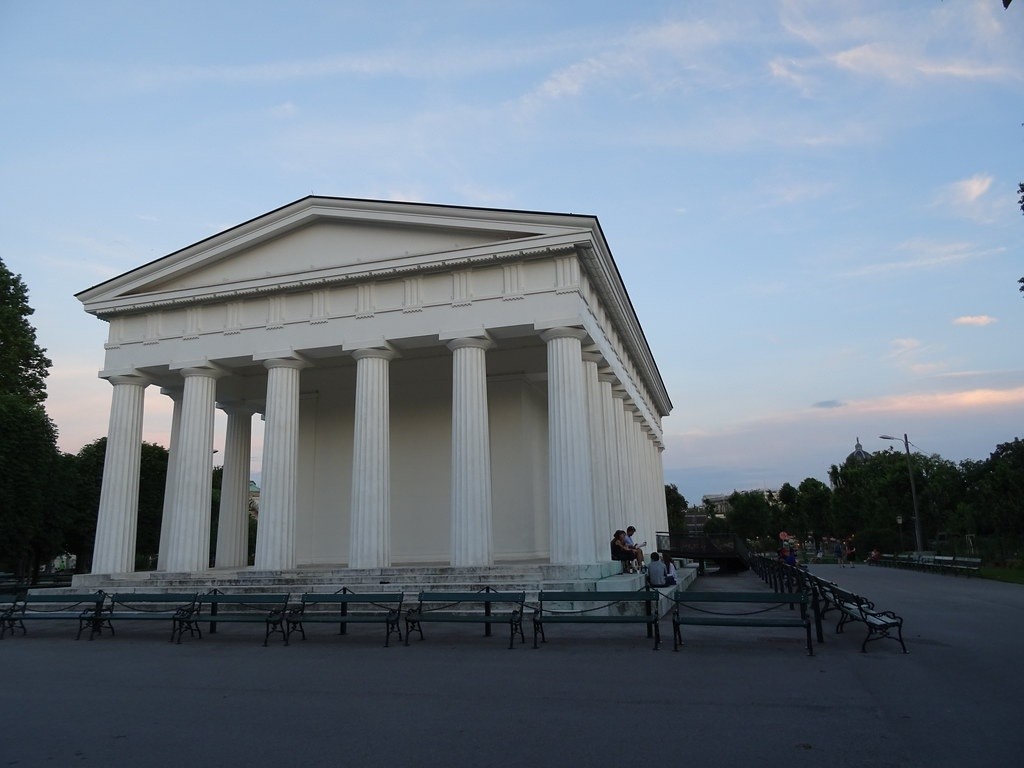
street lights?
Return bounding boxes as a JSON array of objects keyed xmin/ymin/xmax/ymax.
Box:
[{"xmin": 878, "ymin": 432, "xmax": 923, "ymax": 553}]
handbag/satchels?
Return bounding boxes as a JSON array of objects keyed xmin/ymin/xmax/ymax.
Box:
[{"xmin": 847, "ymin": 546, "xmax": 851, "ymax": 555}]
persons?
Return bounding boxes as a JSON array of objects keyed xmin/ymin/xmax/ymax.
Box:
[
  {"xmin": 863, "ymin": 548, "xmax": 880, "ymax": 566},
  {"xmin": 834, "ymin": 539, "xmax": 856, "ymax": 567},
  {"xmin": 784, "ymin": 554, "xmax": 809, "ymax": 572},
  {"xmin": 646, "ymin": 552, "xmax": 677, "ymax": 588},
  {"xmin": 611, "ymin": 526, "xmax": 648, "ymax": 574}
]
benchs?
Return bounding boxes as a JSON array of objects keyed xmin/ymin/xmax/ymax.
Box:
[
  {"xmin": 880, "ymin": 554, "xmax": 896, "ymax": 568},
  {"xmin": 404, "ymin": 590, "xmax": 527, "ymax": 650},
  {"xmin": 178, "ymin": 593, "xmax": 291, "ymax": 648},
  {"xmin": 88, "ymin": 592, "xmax": 195, "ymax": 643},
  {"xmin": 284, "ymin": 593, "xmax": 404, "ymax": 646},
  {"xmin": 869, "ymin": 554, "xmax": 881, "ymax": 567},
  {"xmin": 919, "ymin": 556, "xmax": 937, "ymax": 572},
  {"xmin": 751, "ymin": 554, "xmax": 909, "ymax": 656},
  {"xmin": 951, "ymin": 556, "xmax": 983, "ymax": 579},
  {"xmin": 928, "ymin": 556, "xmax": 956, "ymax": 576},
  {"xmin": 671, "ymin": 588, "xmax": 816, "ymax": 657},
  {"xmin": 893, "ymin": 554, "xmax": 909, "ymax": 569},
  {"xmin": 907, "ymin": 555, "xmax": 924, "ymax": 571},
  {"xmin": 4, "ymin": 594, "xmax": 109, "ymax": 641},
  {"xmin": 532, "ymin": 588, "xmax": 663, "ymax": 651}
]
